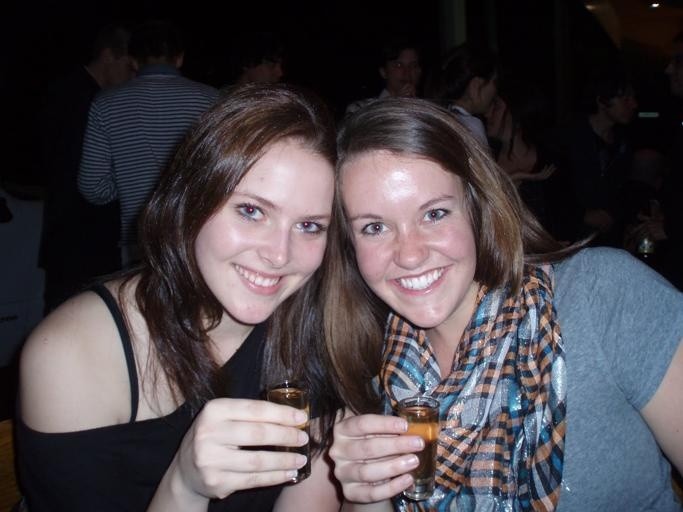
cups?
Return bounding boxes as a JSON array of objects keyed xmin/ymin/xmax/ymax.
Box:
[
  {"xmin": 267, "ymin": 379, "xmax": 312, "ymax": 483},
  {"xmin": 396, "ymin": 397, "xmax": 440, "ymax": 501}
]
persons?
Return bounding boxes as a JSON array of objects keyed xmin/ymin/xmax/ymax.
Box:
[
  {"xmin": 17, "ymin": 80, "xmax": 343, "ymax": 512},
  {"xmin": 319, "ymin": 96, "xmax": 681, "ymax": 512},
  {"xmin": 2, "ymin": 2, "xmax": 681, "ymax": 427}
]
